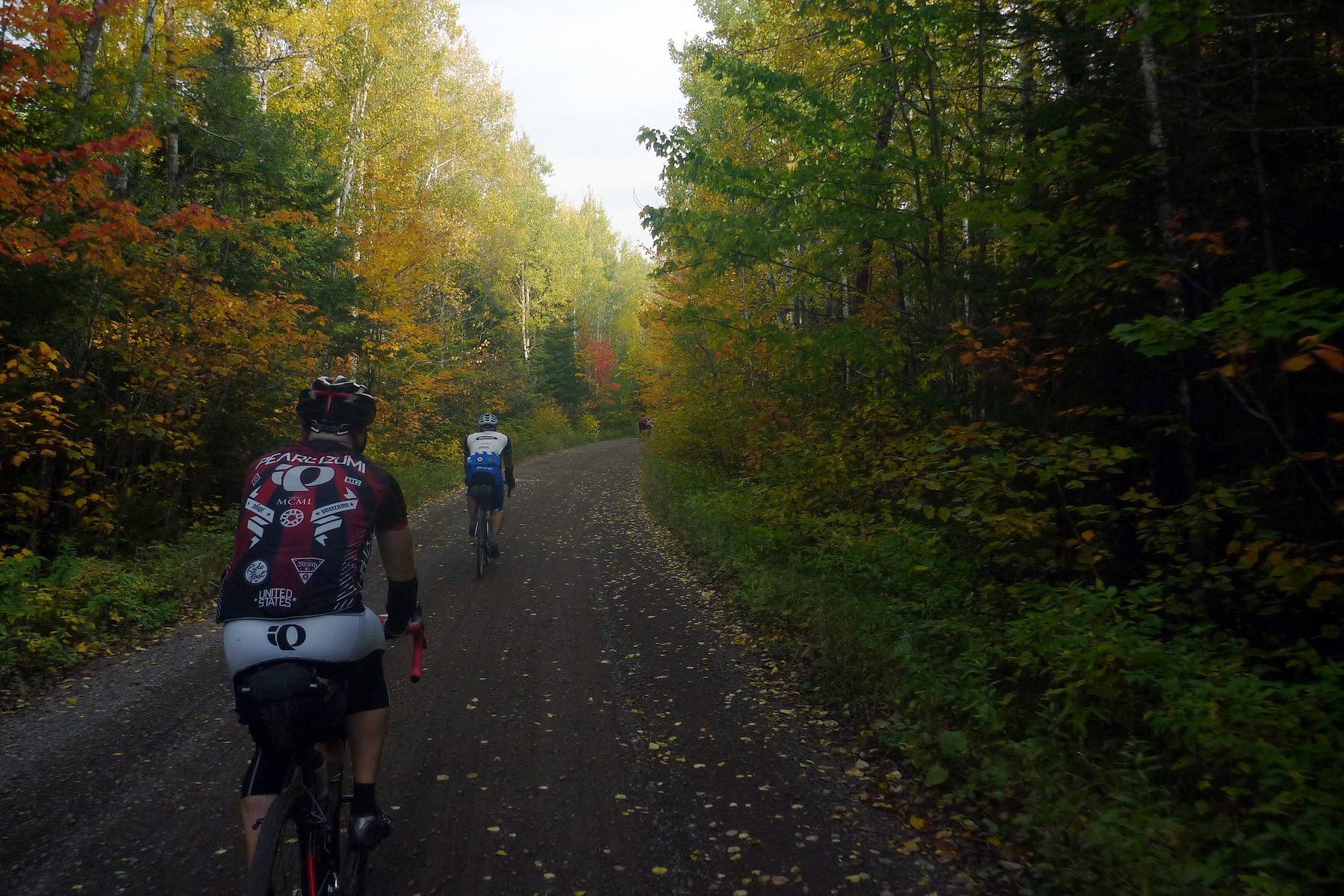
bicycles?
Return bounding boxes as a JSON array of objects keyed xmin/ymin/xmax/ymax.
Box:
[
  {"xmin": 640, "ymin": 423, "xmax": 651, "ymax": 437},
  {"xmin": 247, "ymin": 614, "xmax": 427, "ymax": 896},
  {"xmin": 465, "ymin": 477, "xmax": 512, "ymax": 573}
]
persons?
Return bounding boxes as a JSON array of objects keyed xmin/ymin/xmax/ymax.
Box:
[
  {"xmin": 213, "ymin": 378, "xmax": 419, "ymax": 896},
  {"xmin": 638, "ymin": 414, "xmax": 652, "ymax": 440},
  {"xmin": 463, "ymin": 414, "xmax": 515, "ymax": 557}
]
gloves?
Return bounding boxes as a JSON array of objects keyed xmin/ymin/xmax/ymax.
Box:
[{"xmin": 505, "ymin": 476, "xmax": 515, "ymax": 489}]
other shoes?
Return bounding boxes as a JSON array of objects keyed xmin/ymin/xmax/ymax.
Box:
[
  {"xmin": 489, "ymin": 539, "xmax": 499, "ymax": 558},
  {"xmin": 469, "ymin": 522, "xmax": 477, "ymax": 536},
  {"xmin": 347, "ymin": 810, "xmax": 392, "ymax": 851}
]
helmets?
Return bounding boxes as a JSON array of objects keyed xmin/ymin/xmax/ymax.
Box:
[
  {"xmin": 295, "ymin": 375, "xmax": 376, "ymax": 430},
  {"xmin": 478, "ymin": 413, "xmax": 497, "ymax": 429}
]
